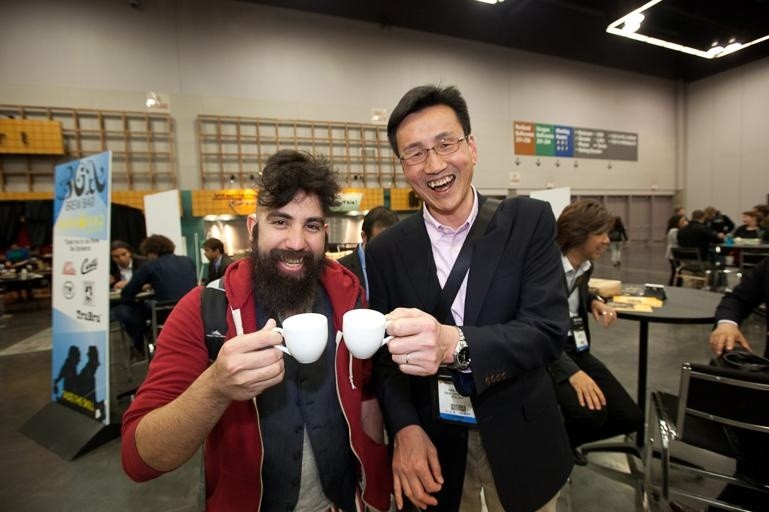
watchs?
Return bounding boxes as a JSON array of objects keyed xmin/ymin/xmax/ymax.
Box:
[{"xmin": 448, "ymin": 328, "xmax": 471, "ymax": 370}]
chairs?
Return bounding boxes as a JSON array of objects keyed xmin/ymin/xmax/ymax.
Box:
[
  {"xmin": 120, "ymin": 300, "xmax": 180, "ymax": 381},
  {"xmin": 642, "ymin": 359, "xmax": 769, "ymax": 512},
  {"xmin": 671, "ymin": 248, "xmax": 719, "ymax": 288}
]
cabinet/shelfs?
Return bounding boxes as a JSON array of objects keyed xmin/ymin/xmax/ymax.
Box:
[{"xmin": 568, "ymin": 190, "xmax": 679, "ymax": 244}]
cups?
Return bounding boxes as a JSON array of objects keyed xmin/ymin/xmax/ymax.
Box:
[
  {"xmin": 271, "ymin": 313, "xmax": 328, "ymax": 364},
  {"xmin": 343, "ymin": 308, "xmax": 395, "ymax": 360}
]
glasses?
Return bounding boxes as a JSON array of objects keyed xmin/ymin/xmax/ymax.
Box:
[{"xmin": 397, "ymin": 135, "xmax": 469, "ymax": 167}]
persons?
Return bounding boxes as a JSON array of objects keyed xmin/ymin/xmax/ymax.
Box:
[
  {"xmin": 5, "ymin": 241, "xmax": 33, "ymax": 301},
  {"xmin": 607, "ymin": 215, "xmax": 629, "ymax": 266},
  {"xmin": 109, "ymin": 240, "xmax": 143, "ymax": 292},
  {"xmin": 203, "ymin": 238, "xmax": 234, "ymax": 284},
  {"xmin": 338, "ymin": 206, "xmax": 398, "ymax": 302},
  {"xmin": 546, "ymin": 199, "xmax": 638, "ymax": 466},
  {"xmin": 709, "ymin": 259, "xmax": 769, "ymax": 358},
  {"xmin": 114, "ymin": 234, "xmax": 197, "ymax": 350},
  {"xmin": 121, "ymin": 150, "xmax": 393, "ymax": 512},
  {"xmin": 665, "ymin": 205, "xmax": 769, "ymax": 292},
  {"xmin": 364, "ymin": 85, "xmax": 574, "ymax": 511}
]
